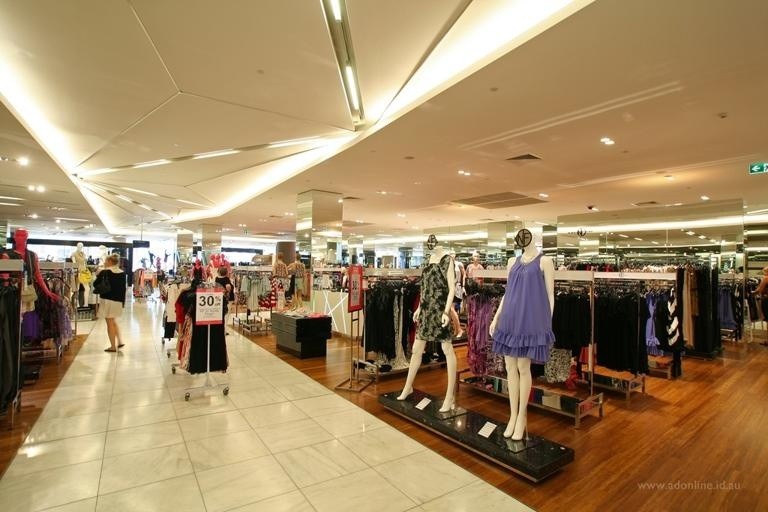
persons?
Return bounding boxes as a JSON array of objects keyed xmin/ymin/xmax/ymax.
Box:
[
  {"xmin": 465, "ymin": 250, "xmax": 486, "ymax": 286},
  {"xmin": 488, "ymin": 232, "xmax": 558, "ymax": 442},
  {"xmin": 0, "ymin": 228, "xmax": 61, "ymax": 302},
  {"xmin": 751, "ymin": 267, "xmax": 768, "ymax": 346},
  {"xmin": 397, "ymin": 245, "xmax": 461, "ymax": 413},
  {"xmin": 215, "ymin": 268, "xmax": 234, "ymax": 335},
  {"xmin": 558, "ymin": 254, "xmax": 573, "ymax": 270},
  {"xmin": 180, "ymin": 266, "xmax": 190, "ymax": 276},
  {"xmin": 449, "ymin": 250, "xmax": 466, "ymax": 338},
  {"xmin": 93, "ymin": 253, "xmax": 126, "ymax": 352},
  {"xmin": 287, "ymin": 253, "xmax": 306, "ymax": 307},
  {"xmin": 190, "ymin": 253, "xmax": 230, "ymax": 282},
  {"xmin": 272, "ymin": 252, "xmax": 288, "ymax": 310},
  {"xmin": 71, "ymin": 242, "xmax": 92, "ymax": 307}
]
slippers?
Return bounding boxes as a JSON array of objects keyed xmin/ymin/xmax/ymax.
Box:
[{"xmin": 104, "ymin": 343, "xmax": 126, "ymax": 352}]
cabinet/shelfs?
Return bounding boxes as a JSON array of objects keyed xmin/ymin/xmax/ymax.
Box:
[{"xmin": 270, "ymin": 308, "xmax": 334, "ymax": 357}]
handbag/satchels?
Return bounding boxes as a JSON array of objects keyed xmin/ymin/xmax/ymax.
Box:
[
  {"xmin": 21, "ymin": 285, "xmax": 38, "ymax": 303},
  {"xmin": 93, "ymin": 270, "xmax": 113, "ymax": 295}
]
